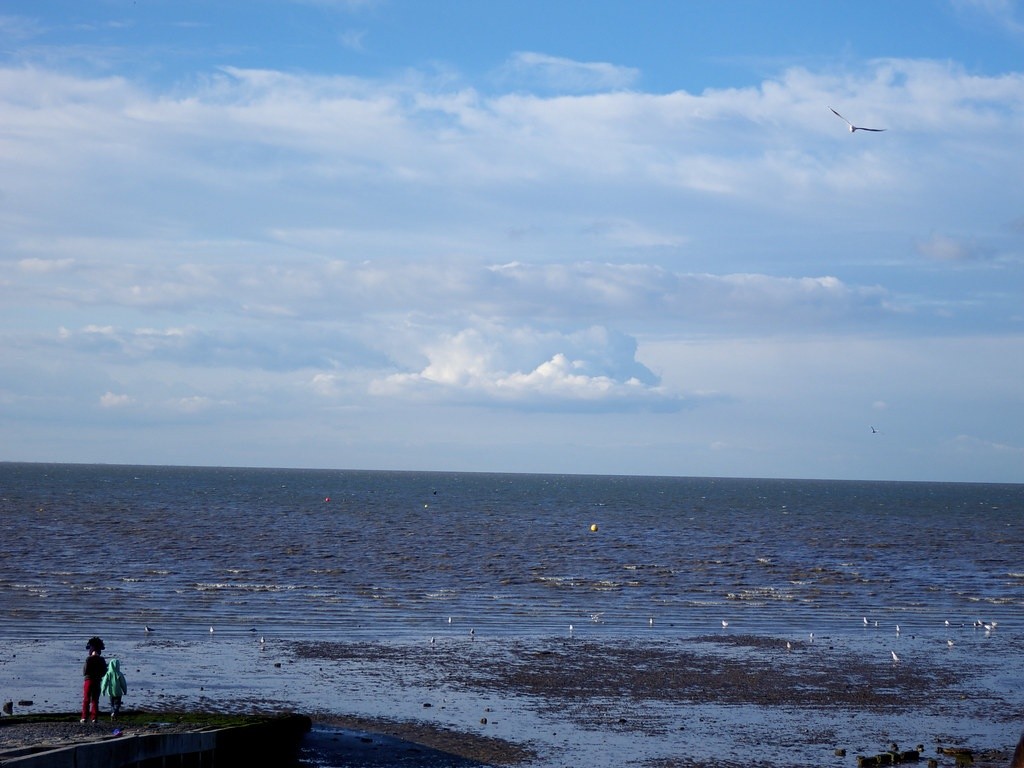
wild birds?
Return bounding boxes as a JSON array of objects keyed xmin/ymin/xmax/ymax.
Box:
[
  {"xmin": 826, "ymin": 106, "xmax": 889, "ymax": 133},
  {"xmin": 569, "ymin": 523, "xmax": 999, "ymax": 663},
  {"xmin": 143, "ymin": 617, "xmax": 477, "ymax": 645}
]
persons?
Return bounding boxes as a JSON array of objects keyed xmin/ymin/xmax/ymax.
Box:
[
  {"xmin": 101, "ymin": 659, "xmax": 128, "ymax": 720},
  {"xmin": 80, "ymin": 636, "xmax": 108, "ymax": 723}
]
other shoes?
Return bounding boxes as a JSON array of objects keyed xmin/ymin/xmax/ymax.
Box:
[
  {"xmin": 111, "ymin": 713, "xmax": 116, "ymax": 717},
  {"xmin": 92, "ymin": 719, "xmax": 98, "ymax": 724},
  {"xmin": 80, "ymin": 719, "xmax": 86, "ymax": 724}
]
